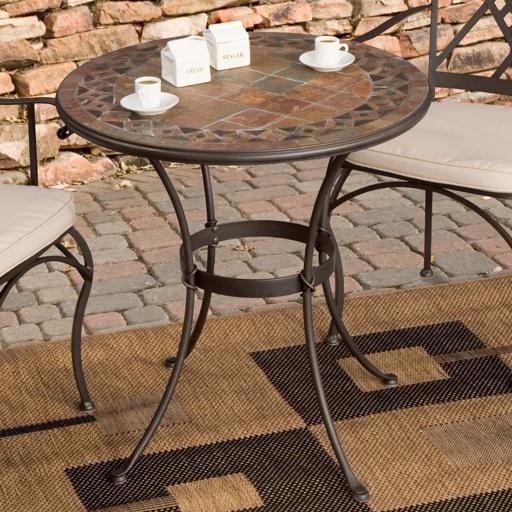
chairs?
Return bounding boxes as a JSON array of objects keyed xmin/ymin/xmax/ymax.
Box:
[
  {"xmin": 0, "ymin": 99, "xmax": 95, "ymax": 412},
  {"xmin": 348, "ymin": 0, "xmax": 512, "ymax": 280}
]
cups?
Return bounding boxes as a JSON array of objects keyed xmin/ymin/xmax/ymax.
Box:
[
  {"xmin": 134, "ymin": 76, "xmax": 161, "ymax": 108},
  {"xmin": 315, "ymin": 36, "xmax": 348, "ymax": 65}
]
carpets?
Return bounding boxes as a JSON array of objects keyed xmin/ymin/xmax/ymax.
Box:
[{"xmin": 0, "ymin": 273, "xmax": 511, "ymax": 511}]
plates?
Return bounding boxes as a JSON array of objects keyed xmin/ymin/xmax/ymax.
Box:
[
  {"xmin": 120, "ymin": 92, "xmax": 179, "ymax": 116},
  {"xmin": 298, "ymin": 50, "xmax": 356, "ymax": 72}
]
